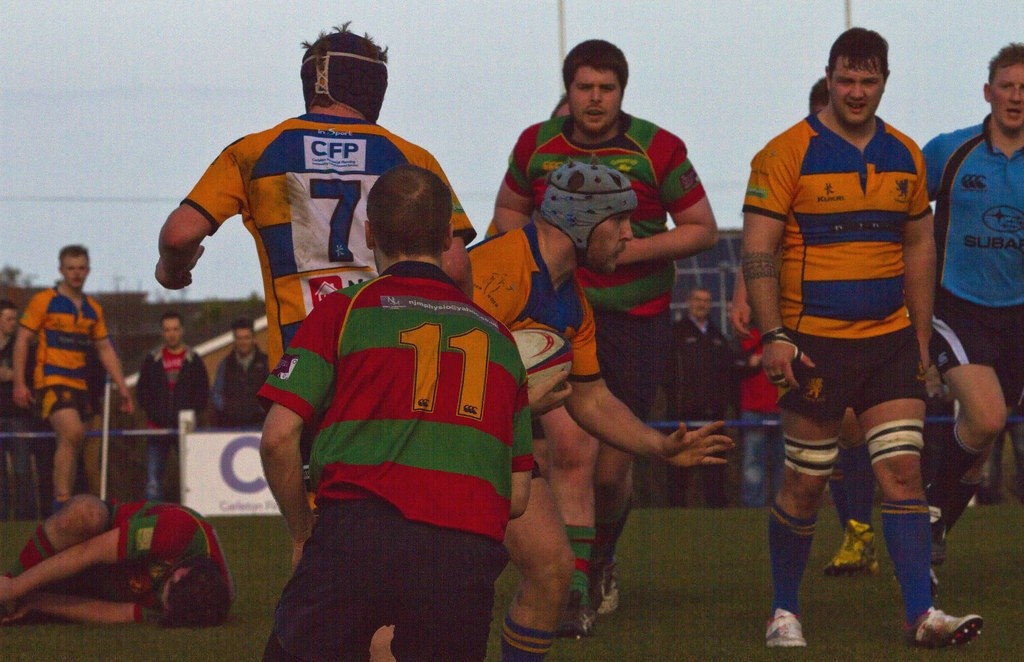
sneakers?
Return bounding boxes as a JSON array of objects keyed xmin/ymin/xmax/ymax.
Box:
[
  {"xmin": 584, "ymin": 554, "xmax": 621, "ymax": 614},
  {"xmin": 902, "ymin": 605, "xmax": 984, "ymax": 648},
  {"xmin": 556, "ymin": 588, "xmax": 595, "ymax": 637},
  {"xmin": 824, "ymin": 518, "xmax": 879, "ymax": 576},
  {"xmin": 764, "ymin": 607, "xmax": 809, "ymax": 646},
  {"xmin": 924, "ymin": 483, "xmax": 949, "ymax": 564}
]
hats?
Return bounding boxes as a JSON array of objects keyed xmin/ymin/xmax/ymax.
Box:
[{"xmin": 540, "ymin": 162, "xmax": 638, "ymax": 252}]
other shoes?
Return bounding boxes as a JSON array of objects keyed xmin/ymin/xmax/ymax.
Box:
[{"xmin": 968, "ymin": 486, "xmax": 1005, "ymax": 508}]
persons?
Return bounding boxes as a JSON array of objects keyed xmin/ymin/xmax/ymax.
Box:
[
  {"xmin": 258, "ymin": 165, "xmax": 534, "ymax": 662},
  {"xmin": 660, "ymin": 285, "xmax": 786, "ymax": 512},
  {"xmin": 495, "ymin": 40, "xmax": 720, "ymax": 615},
  {"xmin": 467, "ymin": 149, "xmax": 737, "ymax": 661},
  {"xmin": 13, "ymin": 245, "xmax": 132, "ymax": 517},
  {"xmin": 209, "ymin": 316, "xmax": 271, "ymax": 428},
  {"xmin": 134, "ymin": 311, "xmax": 209, "ymax": 501},
  {"xmin": 0, "ymin": 300, "xmax": 57, "ymax": 522},
  {"xmin": 742, "ymin": 29, "xmax": 1024, "ymax": 649},
  {"xmin": 0, "ymin": 494, "xmax": 234, "ymax": 630},
  {"xmin": 155, "ymin": 23, "xmax": 476, "ymax": 662}
]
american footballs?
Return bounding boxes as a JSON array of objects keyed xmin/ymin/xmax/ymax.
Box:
[{"xmin": 510, "ymin": 328, "xmax": 574, "ymax": 392}]
